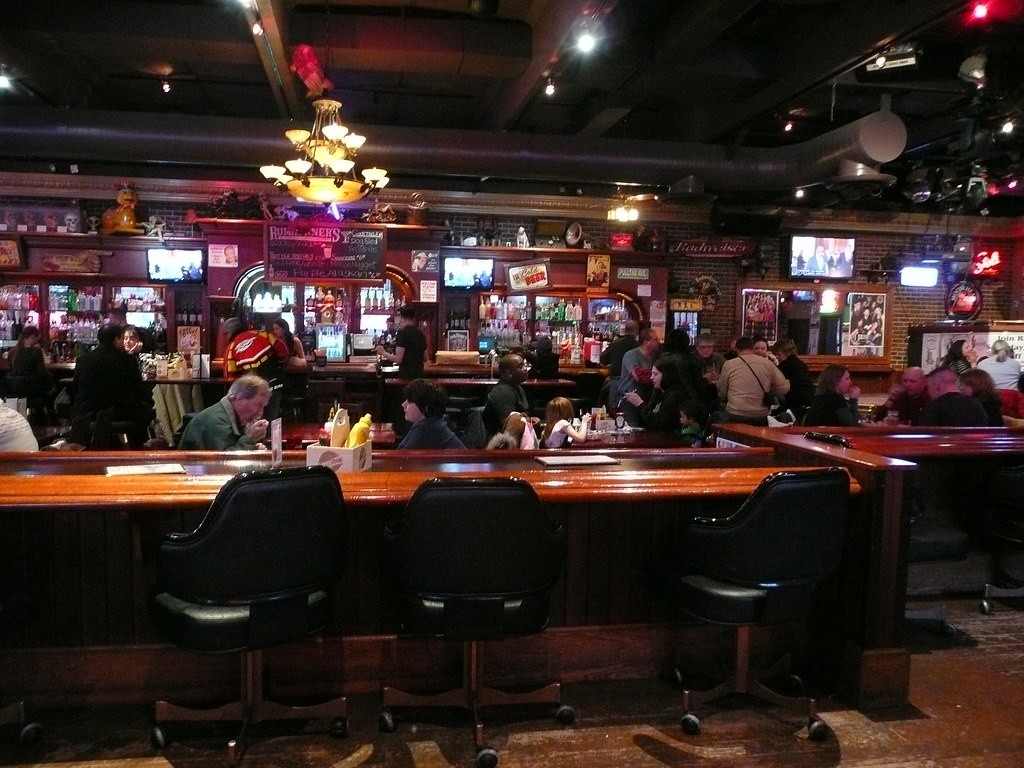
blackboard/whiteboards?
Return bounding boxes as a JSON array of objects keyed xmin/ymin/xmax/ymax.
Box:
[{"xmin": 262, "ymin": 220, "xmax": 386, "ymax": 286}]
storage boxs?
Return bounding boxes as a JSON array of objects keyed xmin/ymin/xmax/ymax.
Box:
[{"xmin": 306, "ymin": 440, "xmax": 372, "ymax": 473}]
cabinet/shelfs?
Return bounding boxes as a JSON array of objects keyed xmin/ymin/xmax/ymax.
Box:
[
  {"xmin": 228, "ymin": 261, "xmax": 423, "ymax": 357},
  {"xmin": 475, "ymin": 283, "xmax": 646, "ymax": 349},
  {"xmin": 0, "ymin": 271, "xmax": 178, "ymax": 354}
]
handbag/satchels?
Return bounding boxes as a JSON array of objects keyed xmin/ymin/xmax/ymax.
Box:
[{"xmin": 762, "ymin": 391, "xmax": 776, "ymax": 407}]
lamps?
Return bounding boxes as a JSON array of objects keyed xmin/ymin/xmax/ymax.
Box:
[
  {"xmin": 607, "ymin": 193, "xmax": 639, "ymax": 222},
  {"xmin": 260, "ymin": 44, "xmax": 390, "ymax": 204}
]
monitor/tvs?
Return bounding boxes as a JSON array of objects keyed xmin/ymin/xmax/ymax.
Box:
[
  {"xmin": 442, "ymin": 256, "xmax": 495, "ymax": 291},
  {"xmin": 146, "ymin": 247, "xmax": 205, "ymax": 282},
  {"xmin": 789, "ymin": 234, "xmax": 857, "ymax": 280}
]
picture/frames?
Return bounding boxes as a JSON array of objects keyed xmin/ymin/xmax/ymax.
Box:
[{"xmin": 945, "ymin": 282, "xmax": 981, "ymax": 320}]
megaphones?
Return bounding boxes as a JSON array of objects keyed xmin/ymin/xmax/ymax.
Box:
[{"xmin": 566, "ymin": 223, "xmax": 612, "ymax": 249}]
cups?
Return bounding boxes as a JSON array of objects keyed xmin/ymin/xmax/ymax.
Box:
[
  {"xmin": 615, "ymin": 407, "xmax": 625, "ymax": 429},
  {"xmin": 884, "ymin": 410, "xmax": 899, "ymax": 426},
  {"xmin": 156, "ymin": 360, "xmax": 168, "ymax": 377}
]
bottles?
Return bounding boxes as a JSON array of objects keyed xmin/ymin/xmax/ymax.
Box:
[
  {"xmin": 373, "ymin": 290, "xmax": 378, "ymax": 310},
  {"xmin": 443, "ymin": 310, "xmax": 471, "ymax": 338},
  {"xmin": 365, "ymin": 328, "xmax": 368, "ymax": 334},
  {"xmin": 316, "ymin": 287, "xmax": 325, "ymax": 308},
  {"xmin": 364, "ymin": 289, "xmax": 370, "ymax": 310},
  {"xmin": 477, "ymin": 299, "xmax": 629, "ymax": 365},
  {"xmin": 380, "ymin": 329, "xmax": 386, "ymax": 346},
  {"xmin": 324, "ymin": 290, "xmax": 335, "ymax": 307},
  {"xmin": 380, "ymin": 290, "xmax": 385, "ymax": 310},
  {"xmin": 341, "ymin": 413, "xmax": 372, "ymax": 448},
  {"xmin": 0, "ymin": 288, "xmax": 151, "ymax": 344},
  {"xmin": 372, "ymin": 329, "xmax": 378, "ymax": 345},
  {"xmin": 176, "ymin": 300, "xmax": 202, "ymax": 325}
]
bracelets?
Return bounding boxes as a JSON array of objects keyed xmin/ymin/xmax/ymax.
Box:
[{"xmin": 887, "ymin": 397, "xmax": 897, "ymax": 402}]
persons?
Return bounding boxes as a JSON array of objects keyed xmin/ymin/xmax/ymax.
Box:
[
  {"xmin": 631, "ymin": 225, "xmax": 666, "ymax": 252},
  {"xmin": 599, "ymin": 320, "xmax": 818, "ymax": 448},
  {"xmin": 0, "ymin": 307, "xmax": 153, "ymax": 450},
  {"xmin": 796, "ymin": 246, "xmax": 854, "ymax": 276},
  {"xmin": 850, "ymin": 295, "xmax": 883, "ymax": 346},
  {"xmin": 745, "ymin": 293, "xmax": 777, "ymax": 322},
  {"xmin": 587, "ymin": 262, "xmax": 608, "ymax": 286},
  {"xmin": 874, "ymin": 340, "xmax": 1024, "ymax": 428},
  {"xmin": 802, "ymin": 364, "xmax": 861, "ymax": 428},
  {"xmin": 395, "ymin": 378, "xmax": 468, "ymax": 451},
  {"xmin": 481, "ymin": 336, "xmax": 592, "ymax": 449},
  {"xmin": 471, "ymin": 218, "xmax": 502, "ymax": 247},
  {"xmin": 178, "ymin": 375, "xmax": 269, "ymax": 451},
  {"xmin": 372, "ymin": 308, "xmax": 428, "ymax": 421},
  {"xmin": 224, "ymin": 316, "xmax": 307, "ymax": 421}
]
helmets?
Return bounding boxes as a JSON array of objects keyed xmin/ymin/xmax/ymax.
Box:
[{"xmin": 223, "ymin": 317, "xmax": 244, "ymax": 338}]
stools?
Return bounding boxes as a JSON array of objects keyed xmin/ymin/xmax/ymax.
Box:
[
  {"xmin": 449, "ymin": 393, "xmax": 480, "ymax": 431},
  {"xmin": 568, "ymin": 395, "xmax": 588, "ymax": 415},
  {"xmin": 88, "ymin": 421, "xmax": 132, "ymax": 450},
  {"xmin": 279, "ymin": 396, "xmax": 305, "ymax": 424}
]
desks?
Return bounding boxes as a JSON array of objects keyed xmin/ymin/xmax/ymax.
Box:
[
  {"xmin": 384, "ymin": 377, "xmax": 576, "ymax": 386},
  {"xmin": 61, "ymin": 376, "xmax": 237, "ymax": 383},
  {"xmin": 571, "ymin": 428, "xmax": 685, "ymax": 447},
  {"xmin": 266, "ymin": 422, "xmax": 396, "ymax": 444},
  {"xmin": 28, "ymin": 425, "xmax": 73, "ymax": 444},
  {"xmin": 0, "ymin": 465, "xmax": 863, "ymax": 508},
  {"xmin": 843, "ymin": 432, "xmax": 1024, "ymax": 455}
]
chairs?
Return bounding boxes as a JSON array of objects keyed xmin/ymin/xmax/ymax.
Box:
[
  {"xmin": 458, "ymin": 412, "xmax": 490, "ymax": 449},
  {"xmin": 994, "ymin": 389, "xmax": 1024, "ymax": 419},
  {"xmin": 978, "ymin": 464, "xmax": 1024, "ymax": 614},
  {"xmin": 380, "ymin": 474, "xmax": 576, "ymax": 768},
  {"xmin": 153, "ymin": 463, "xmax": 350, "ymax": 768},
  {"xmin": 666, "ymin": 467, "xmax": 852, "ymax": 743}
]
